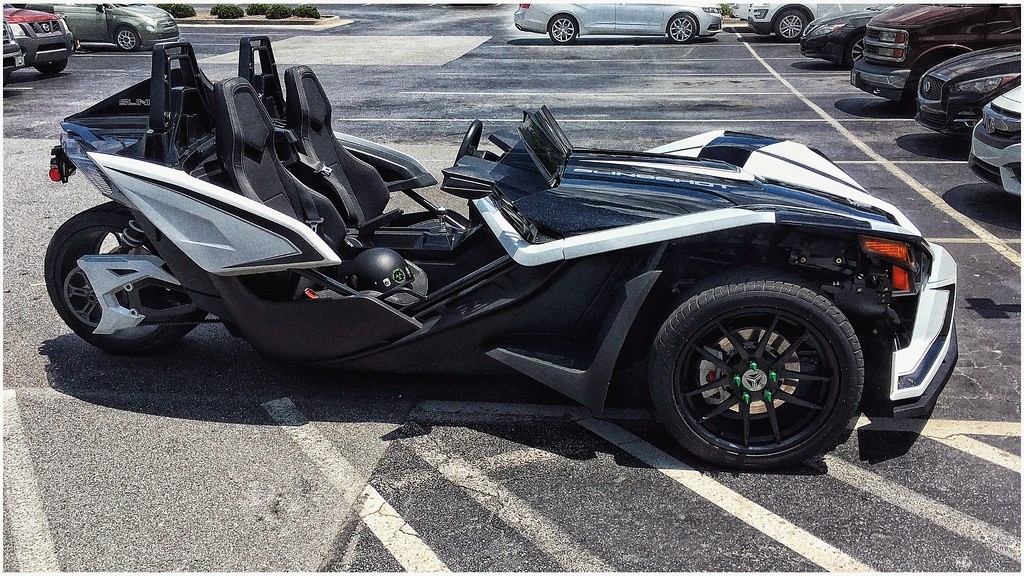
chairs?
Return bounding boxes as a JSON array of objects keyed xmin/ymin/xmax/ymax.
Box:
[
  {"xmin": 284, "ymin": 65, "xmax": 469, "ymax": 235},
  {"xmin": 213, "ymin": 77, "xmax": 428, "ymax": 308}
]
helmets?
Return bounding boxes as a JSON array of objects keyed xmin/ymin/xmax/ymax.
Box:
[{"xmin": 347, "ymin": 247, "xmax": 415, "ymax": 293}]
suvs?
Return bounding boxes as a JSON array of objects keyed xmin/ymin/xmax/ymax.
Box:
[
  {"xmin": 850, "ymin": 4, "xmax": 1021, "ymax": 114},
  {"xmin": 3, "ymin": 3, "xmax": 74, "ymax": 75}
]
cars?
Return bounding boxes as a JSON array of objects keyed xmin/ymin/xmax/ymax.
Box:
[
  {"xmin": 801, "ymin": 4, "xmax": 902, "ymax": 69},
  {"xmin": 514, "ymin": 4, "xmax": 723, "ymax": 45},
  {"xmin": 3, "ymin": 17, "xmax": 25, "ymax": 87},
  {"xmin": 967, "ymin": 85, "xmax": 1021, "ymax": 197},
  {"xmin": 914, "ymin": 42, "xmax": 1021, "ymax": 144},
  {"xmin": 747, "ymin": 4, "xmax": 892, "ymax": 43},
  {"xmin": 25, "ymin": 4, "xmax": 180, "ymax": 52}
]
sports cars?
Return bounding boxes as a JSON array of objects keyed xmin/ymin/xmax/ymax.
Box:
[{"xmin": 44, "ymin": 34, "xmax": 959, "ymax": 474}]
